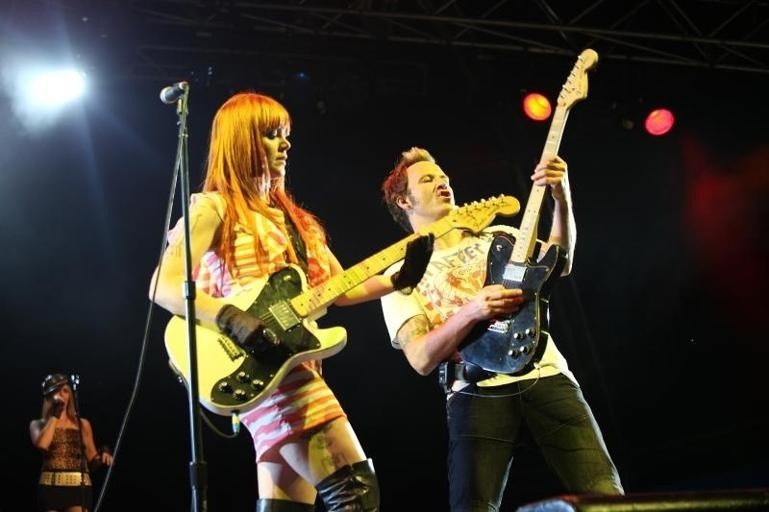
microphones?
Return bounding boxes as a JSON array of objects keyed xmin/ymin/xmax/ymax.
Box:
[
  {"xmin": 56, "ymin": 378, "xmax": 80, "ymax": 387},
  {"xmin": 159, "ymin": 82, "xmax": 188, "ymax": 105}
]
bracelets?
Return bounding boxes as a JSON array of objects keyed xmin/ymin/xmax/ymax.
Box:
[
  {"xmin": 90, "ymin": 453, "xmax": 105, "ymax": 465},
  {"xmin": 49, "ymin": 398, "xmax": 65, "ymax": 418}
]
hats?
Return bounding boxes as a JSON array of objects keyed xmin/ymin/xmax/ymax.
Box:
[{"xmin": 42, "ymin": 373, "xmax": 69, "ymax": 395}]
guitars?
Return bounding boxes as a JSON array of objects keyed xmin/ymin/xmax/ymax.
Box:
[
  {"xmin": 164, "ymin": 193, "xmax": 520, "ymax": 416},
  {"xmin": 457, "ymin": 49, "xmax": 599, "ymax": 376}
]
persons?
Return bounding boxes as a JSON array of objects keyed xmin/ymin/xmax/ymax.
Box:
[
  {"xmin": 27, "ymin": 370, "xmax": 115, "ymax": 511},
  {"xmin": 147, "ymin": 94, "xmax": 435, "ymax": 509},
  {"xmin": 376, "ymin": 143, "xmax": 626, "ymax": 510}
]
